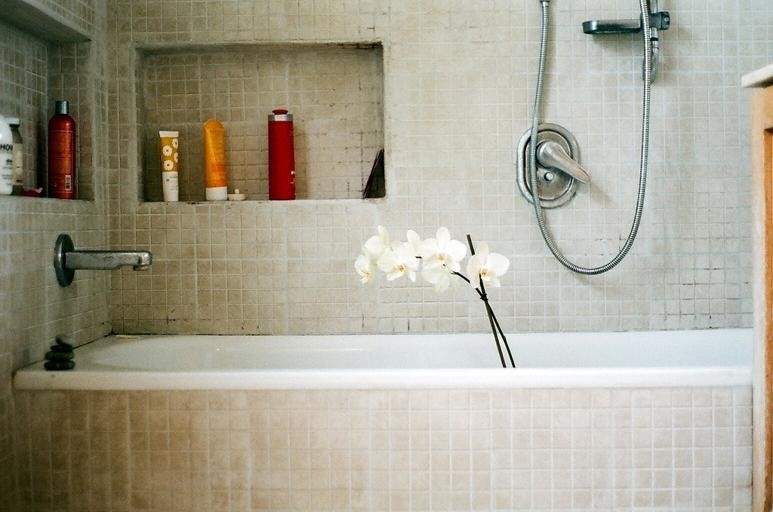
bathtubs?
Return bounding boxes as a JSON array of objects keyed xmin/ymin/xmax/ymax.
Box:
[{"xmin": 15, "ymin": 328, "xmax": 756, "ymax": 391}]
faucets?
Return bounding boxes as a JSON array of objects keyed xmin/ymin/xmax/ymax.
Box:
[{"xmin": 65, "ymin": 249, "xmax": 152, "ymax": 274}]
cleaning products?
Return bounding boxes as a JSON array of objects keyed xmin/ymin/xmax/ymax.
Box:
[{"xmin": 46, "ymin": 98, "xmax": 76, "ymax": 200}]
